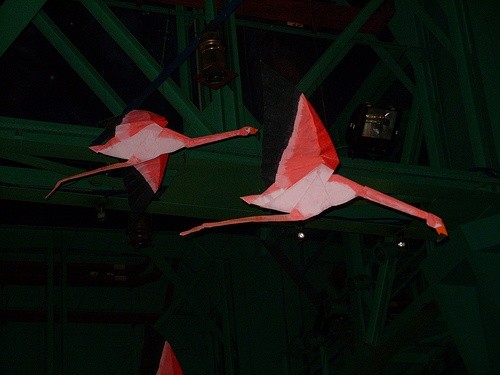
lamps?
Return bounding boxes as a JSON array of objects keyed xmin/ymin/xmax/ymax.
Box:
[
  {"xmin": 344, "ymin": 103, "xmax": 400, "ymax": 156},
  {"xmin": 124, "ymin": 197, "xmax": 154, "ymax": 253},
  {"xmin": 196, "ymin": 28, "xmax": 240, "ymax": 93}
]
